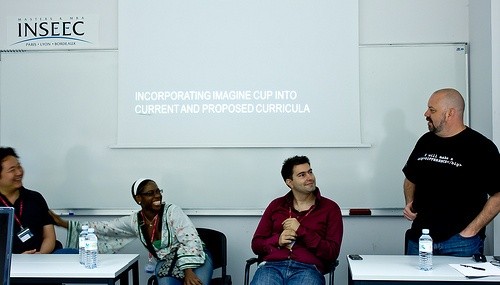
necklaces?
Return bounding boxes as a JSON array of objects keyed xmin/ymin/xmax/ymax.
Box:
[{"xmin": 145, "ymin": 216, "xmax": 157, "ymax": 227}]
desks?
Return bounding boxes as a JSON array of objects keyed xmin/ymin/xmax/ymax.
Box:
[
  {"xmin": 9, "ymin": 254, "xmax": 140, "ymax": 285},
  {"xmin": 346, "ymin": 254, "xmax": 500, "ymax": 285}
]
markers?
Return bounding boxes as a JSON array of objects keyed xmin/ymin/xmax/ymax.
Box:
[{"xmin": 55, "ymin": 213, "xmax": 74, "ymax": 216}]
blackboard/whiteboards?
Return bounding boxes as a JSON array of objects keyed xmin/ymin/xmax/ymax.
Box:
[{"xmin": 0, "ymin": 42, "xmax": 469, "ymax": 216}]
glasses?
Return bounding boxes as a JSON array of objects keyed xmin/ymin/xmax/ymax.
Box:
[{"xmin": 141, "ymin": 189, "xmax": 163, "ymax": 196}]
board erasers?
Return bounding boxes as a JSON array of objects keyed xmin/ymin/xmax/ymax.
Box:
[{"xmin": 350, "ymin": 209, "xmax": 371, "ymax": 215}]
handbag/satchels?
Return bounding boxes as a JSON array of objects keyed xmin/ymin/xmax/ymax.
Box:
[{"xmin": 156, "ymin": 249, "xmax": 186, "ymax": 280}]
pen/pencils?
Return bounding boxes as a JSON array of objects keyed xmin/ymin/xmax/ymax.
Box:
[{"xmin": 460, "ymin": 264, "xmax": 485, "ymax": 271}]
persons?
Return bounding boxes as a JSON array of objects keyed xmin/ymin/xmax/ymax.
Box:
[
  {"xmin": 48, "ymin": 178, "xmax": 213, "ymax": 285},
  {"xmin": 250, "ymin": 155, "xmax": 343, "ymax": 285},
  {"xmin": 402, "ymin": 88, "xmax": 500, "ymax": 258},
  {"xmin": 0, "ymin": 147, "xmax": 62, "ymax": 285}
]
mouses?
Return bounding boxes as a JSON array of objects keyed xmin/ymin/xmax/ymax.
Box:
[{"xmin": 473, "ymin": 254, "xmax": 486, "ymax": 262}]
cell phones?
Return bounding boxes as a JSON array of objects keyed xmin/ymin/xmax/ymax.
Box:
[{"xmin": 349, "ymin": 255, "xmax": 362, "ymax": 260}]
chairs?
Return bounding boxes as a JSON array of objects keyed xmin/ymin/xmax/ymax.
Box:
[
  {"xmin": 147, "ymin": 227, "xmax": 233, "ymax": 285},
  {"xmin": 244, "ymin": 255, "xmax": 340, "ymax": 285}
]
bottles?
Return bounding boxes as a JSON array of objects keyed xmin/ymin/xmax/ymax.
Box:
[
  {"xmin": 85, "ymin": 228, "xmax": 97, "ymax": 269},
  {"xmin": 79, "ymin": 225, "xmax": 89, "ymax": 265},
  {"xmin": 418, "ymin": 229, "xmax": 433, "ymax": 271}
]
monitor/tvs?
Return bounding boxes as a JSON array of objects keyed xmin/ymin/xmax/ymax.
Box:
[{"xmin": 0, "ymin": 207, "xmax": 14, "ymax": 285}]
